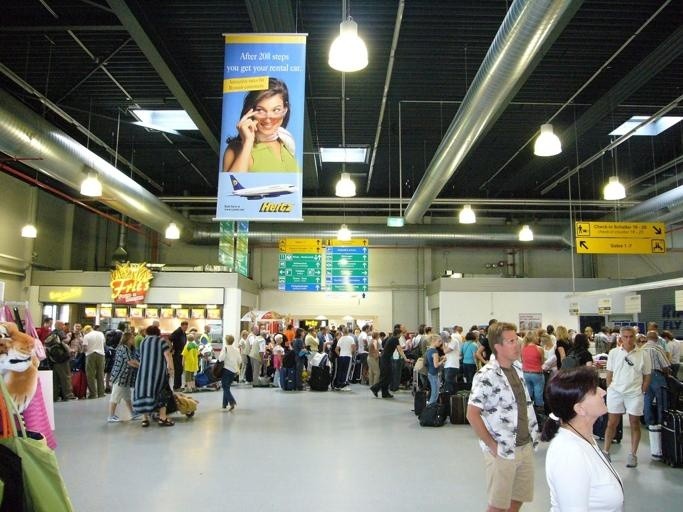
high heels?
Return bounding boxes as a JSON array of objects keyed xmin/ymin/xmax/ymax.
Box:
[{"xmin": 229, "ymin": 400, "xmax": 237, "ymax": 411}]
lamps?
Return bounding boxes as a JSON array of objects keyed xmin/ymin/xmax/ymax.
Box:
[
  {"xmin": 337, "ymin": 206, "xmax": 353, "ymax": 243},
  {"xmin": 603, "ymin": 90, "xmax": 626, "ymax": 202},
  {"xmin": 456, "ymin": 157, "xmax": 476, "ymax": 225},
  {"xmin": 532, "ymin": 67, "xmax": 561, "ymax": 158},
  {"xmin": 334, "ymin": 97, "xmax": 356, "ymax": 199},
  {"xmin": 19, "ymin": 186, "xmax": 38, "ymax": 239},
  {"xmin": 518, "ymin": 184, "xmax": 533, "ymax": 242},
  {"xmin": 324, "ymin": 2, "xmax": 370, "ymax": 74},
  {"xmin": 79, "ymin": 77, "xmax": 102, "ymax": 196},
  {"xmin": 163, "ymin": 135, "xmax": 181, "ymax": 240}
]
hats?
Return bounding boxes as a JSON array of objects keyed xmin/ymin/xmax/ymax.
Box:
[{"xmin": 440, "ymin": 331, "xmax": 449, "ymax": 341}]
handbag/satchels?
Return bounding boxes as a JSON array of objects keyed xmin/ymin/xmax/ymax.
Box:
[
  {"xmin": 648, "ymin": 424, "xmax": 662, "ymax": 461},
  {"xmin": 71, "ymin": 353, "xmax": 85, "ymax": 368},
  {"xmin": 195, "ymin": 360, "xmax": 223, "ymax": 387},
  {"xmin": 414, "ymin": 357, "xmax": 428, "ymax": 377},
  {"xmin": 33, "ymin": 338, "xmax": 46, "ymax": 359},
  {"xmin": 0, "ymin": 431, "xmax": 74, "ymax": 512}
]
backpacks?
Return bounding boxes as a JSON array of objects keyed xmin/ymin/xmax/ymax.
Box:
[
  {"xmin": 283, "ymin": 350, "xmax": 294, "ymax": 368},
  {"xmin": 418, "ymin": 403, "xmax": 445, "ymax": 427},
  {"xmin": 44, "ymin": 333, "xmax": 70, "ymax": 364}
]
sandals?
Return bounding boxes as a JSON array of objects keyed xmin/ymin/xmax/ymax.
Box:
[
  {"xmin": 141, "ymin": 421, "xmax": 149, "ymax": 427},
  {"xmin": 158, "ymin": 418, "xmax": 174, "ymax": 426}
]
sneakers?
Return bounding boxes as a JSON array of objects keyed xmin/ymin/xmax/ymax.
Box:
[
  {"xmin": 625, "ymin": 455, "xmax": 636, "ymax": 467},
  {"xmin": 107, "ymin": 415, "xmax": 123, "ymax": 422},
  {"xmin": 129, "ymin": 413, "xmax": 142, "ymax": 420}
]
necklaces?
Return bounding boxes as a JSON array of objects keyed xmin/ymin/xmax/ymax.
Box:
[{"xmin": 568, "ymin": 422, "xmax": 624, "ymax": 497}]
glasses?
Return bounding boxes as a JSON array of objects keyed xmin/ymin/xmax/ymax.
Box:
[{"xmin": 624, "ymin": 357, "xmax": 632, "ymax": 366}]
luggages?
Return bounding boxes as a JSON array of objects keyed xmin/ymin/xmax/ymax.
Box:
[
  {"xmin": 309, "ymin": 366, "xmax": 328, "ymax": 391},
  {"xmin": 449, "ymin": 394, "xmax": 464, "ymax": 424},
  {"xmin": 592, "ymin": 413, "xmax": 622, "ymax": 443},
  {"xmin": 71, "ymin": 370, "xmax": 87, "ymax": 399},
  {"xmin": 280, "ymin": 368, "xmax": 295, "ymax": 390},
  {"xmin": 660, "ymin": 385, "xmax": 682, "ymax": 468},
  {"xmin": 414, "ymin": 391, "xmax": 425, "ymax": 415}
]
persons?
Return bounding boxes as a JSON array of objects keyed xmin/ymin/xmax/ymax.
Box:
[
  {"xmin": 222, "ymin": 77, "xmax": 300, "ymax": 173},
  {"xmin": 539, "ymin": 366, "xmax": 624, "ymax": 511},
  {"xmin": 239, "ymin": 323, "xmax": 494, "ymax": 406},
  {"xmin": 218, "ymin": 335, "xmax": 242, "ymax": 413},
  {"xmin": 513, "ymin": 320, "xmax": 682, "ymax": 469},
  {"xmin": 466, "ymin": 322, "xmax": 538, "ymax": 511},
  {"xmin": 34, "ymin": 316, "xmax": 215, "ymax": 427}
]
